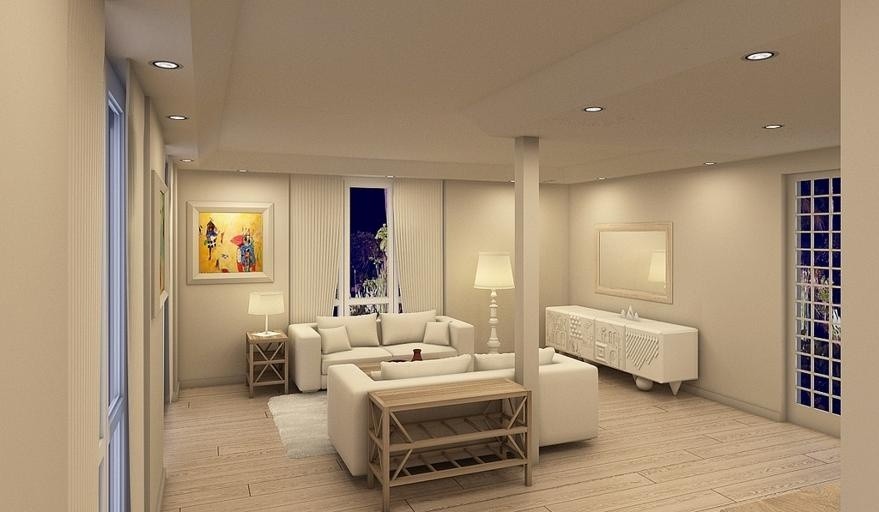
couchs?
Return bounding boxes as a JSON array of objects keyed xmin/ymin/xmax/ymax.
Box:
[
  {"xmin": 327, "ymin": 349, "xmax": 600, "ymax": 481},
  {"xmin": 288, "ymin": 309, "xmax": 475, "ymax": 394}
]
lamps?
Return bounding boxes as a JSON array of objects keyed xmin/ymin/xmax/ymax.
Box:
[
  {"xmin": 472, "ymin": 252, "xmax": 514, "ymax": 353},
  {"xmin": 247, "ymin": 291, "xmax": 285, "ymax": 337}
]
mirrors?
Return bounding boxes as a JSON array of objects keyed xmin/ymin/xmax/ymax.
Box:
[{"xmin": 592, "ymin": 221, "xmax": 673, "ymax": 305}]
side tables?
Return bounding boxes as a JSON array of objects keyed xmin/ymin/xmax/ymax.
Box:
[{"xmin": 245, "ymin": 330, "xmax": 290, "ymax": 399}]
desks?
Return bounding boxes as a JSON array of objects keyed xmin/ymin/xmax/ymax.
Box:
[{"xmin": 365, "ymin": 379, "xmax": 533, "ymax": 511}]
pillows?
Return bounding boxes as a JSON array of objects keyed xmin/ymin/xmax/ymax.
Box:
[
  {"xmin": 423, "ymin": 320, "xmax": 454, "ymax": 346},
  {"xmin": 317, "ymin": 326, "xmax": 352, "ymax": 354}
]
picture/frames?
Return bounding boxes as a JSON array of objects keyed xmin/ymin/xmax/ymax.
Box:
[
  {"xmin": 186, "ymin": 201, "xmax": 274, "ymax": 284},
  {"xmin": 151, "ymin": 168, "xmax": 170, "ymax": 318}
]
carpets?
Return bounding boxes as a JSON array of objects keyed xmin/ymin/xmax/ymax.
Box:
[{"xmin": 265, "ymin": 387, "xmax": 333, "ymax": 460}]
